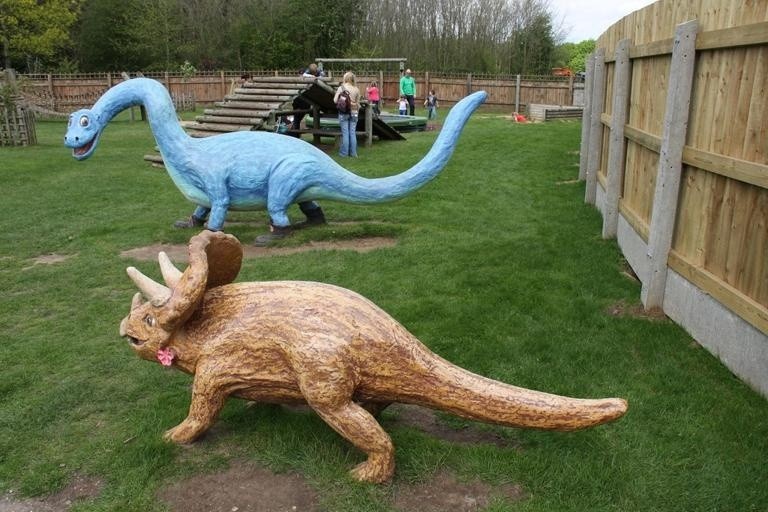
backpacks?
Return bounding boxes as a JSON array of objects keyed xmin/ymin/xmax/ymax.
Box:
[{"xmin": 336, "ymin": 82, "xmax": 352, "ymax": 119}]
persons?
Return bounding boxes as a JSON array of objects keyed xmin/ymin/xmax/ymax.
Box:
[
  {"xmin": 423, "ymin": 90, "xmax": 439, "ymax": 123},
  {"xmin": 365, "ymin": 81, "xmax": 381, "ymax": 116},
  {"xmin": 396, "ymin": 95, "xmax": 410, "ymax": 116},
  {"xmin": 398, "ymin": 68, "xmax": 417, "ymax": 116},
  {"xmin": 332, "ymin": 72, "xmax": 360, "ymax": 159},
  {"xmin": 511, "ymin": 111, "xmax": 528, "ymax": 124},
  {"xmin": 302, "ymin": 64, "xmax": 328, "ymax": 87}
]
